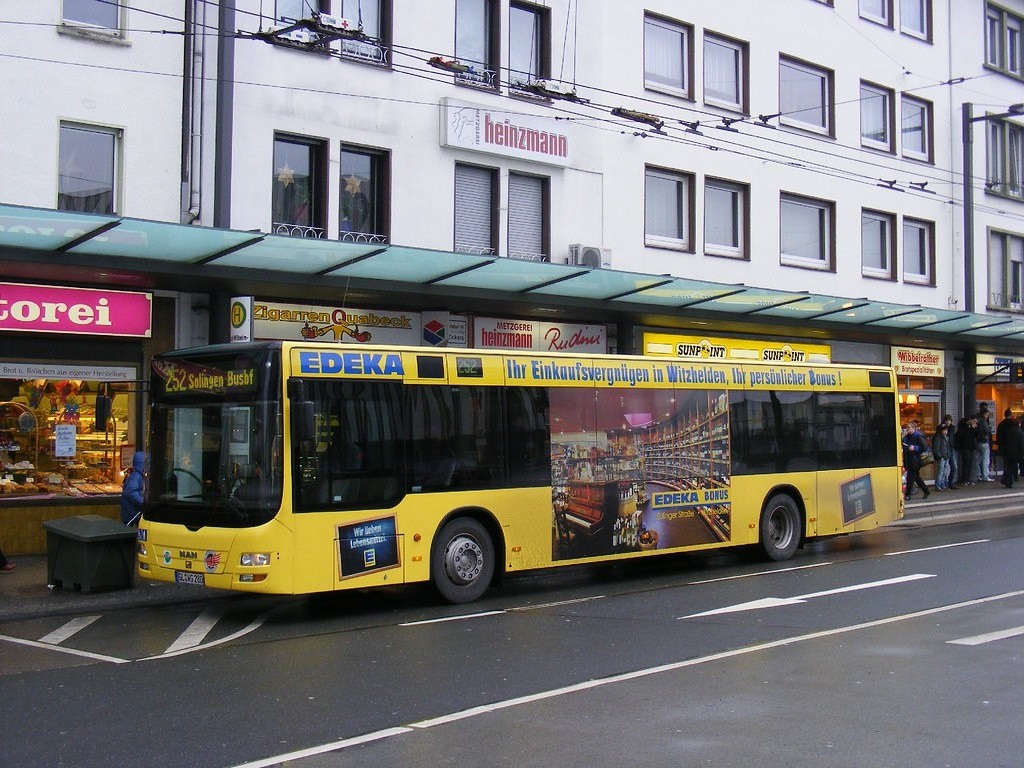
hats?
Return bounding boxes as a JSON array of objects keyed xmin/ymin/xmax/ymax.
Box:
[
  {"xmin": 937, "ymin": 423, "xmax": 947, "ymax": 429},
  {"xmin": 942, "ymin": 414, "xmax": 953, "ymax": 421}
]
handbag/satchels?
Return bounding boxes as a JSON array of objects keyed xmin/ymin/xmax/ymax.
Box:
[{"xmin": 919, "ymin": 448, "xmax": 936, "ymax": 466}]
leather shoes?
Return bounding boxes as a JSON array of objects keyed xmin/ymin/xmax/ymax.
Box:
[{"xmin": 2, "ymin": 561, "xmax": 17, "ymax": 571}]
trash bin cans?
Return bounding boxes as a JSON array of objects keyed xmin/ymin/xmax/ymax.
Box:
[{"xmin": 42, "ymin": 513, "xmax": 140, "ymax": 595}]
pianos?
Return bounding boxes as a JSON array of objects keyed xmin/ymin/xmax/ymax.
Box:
[{"xmin": 555, "ymin": 478, "xmax": 621, "ymax": 554}]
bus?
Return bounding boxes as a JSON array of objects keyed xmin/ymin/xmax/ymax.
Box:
[{"xmin": 95, "ymin": 341, "xmax": 909, "ymax": 606}]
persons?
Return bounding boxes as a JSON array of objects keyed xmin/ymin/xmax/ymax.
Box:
[
  {"xmin": 931, "ymin": 400, "xmax": 996, "ymax": 491},
  {"xmin": 902, "ymin": 421, "xmax": 931, "ymax": 499},
  {"xmin": 120, "ymin": 451, "xmax": 151, "ymax": 526},
  {"xmin": 997, "ymin": 409, "xmax": 1020, "ymax": 489},
  {"xmin": 0, "ymin": 536, "xmax": 18, "ymax": 572},
  {"xmin": 1012, "ymin": 413, "xmax": 1024, "ymax": 483}
]
